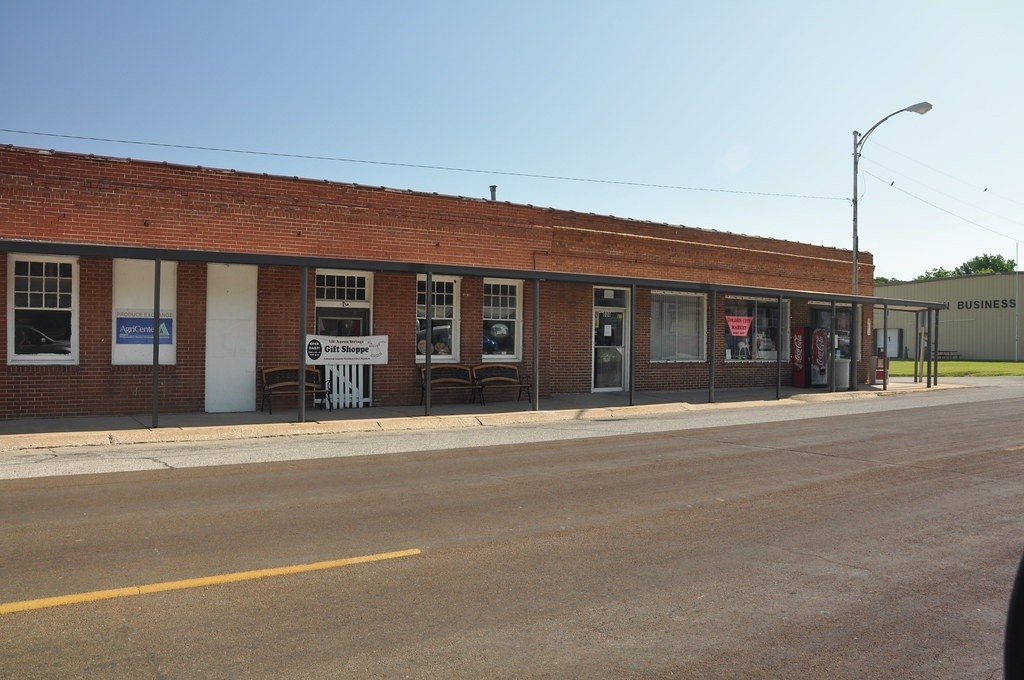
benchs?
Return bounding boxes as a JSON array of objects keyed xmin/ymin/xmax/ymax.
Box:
[
  {"xmin": 259, "ymin": 364, "xmax": 333, "ymax": 416},
  {"xmin": 418, "ymin": 364, "xmax": 485, "ymax": 408},
  {"xmin": 471, "ymin": 364, "xmax": 533, "ymax": 407},
  {"xmin": 933, "ymin": 354, "xmax": 961, "ymax": 360}
]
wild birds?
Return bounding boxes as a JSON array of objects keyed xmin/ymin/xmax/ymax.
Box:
[
  {"xmin": 984, "ymin": 188, "xmax": 987, "ymax": 191},
  {"xmin": 891, "ymin": 181, "xmax": 894, "ymax": 186}
]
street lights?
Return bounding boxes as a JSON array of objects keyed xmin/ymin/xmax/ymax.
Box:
[{"xmin": 851, "ymin": 102, "xmax": 933, "ymax": 392}]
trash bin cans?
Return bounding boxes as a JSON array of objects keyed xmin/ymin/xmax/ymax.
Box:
[{"xmin": 830, "ymin": 358, "xmax": 851, "ymax": 391}]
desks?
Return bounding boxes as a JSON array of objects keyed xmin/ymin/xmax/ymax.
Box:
[{"xmin": 932, "ymin": 350, "xmax": 958, "ymax": 360}]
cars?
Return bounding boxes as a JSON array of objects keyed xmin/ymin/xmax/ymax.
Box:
[{"xmin": 16, "ymin": 324, "xmax": 70, "ymax": 354}]
